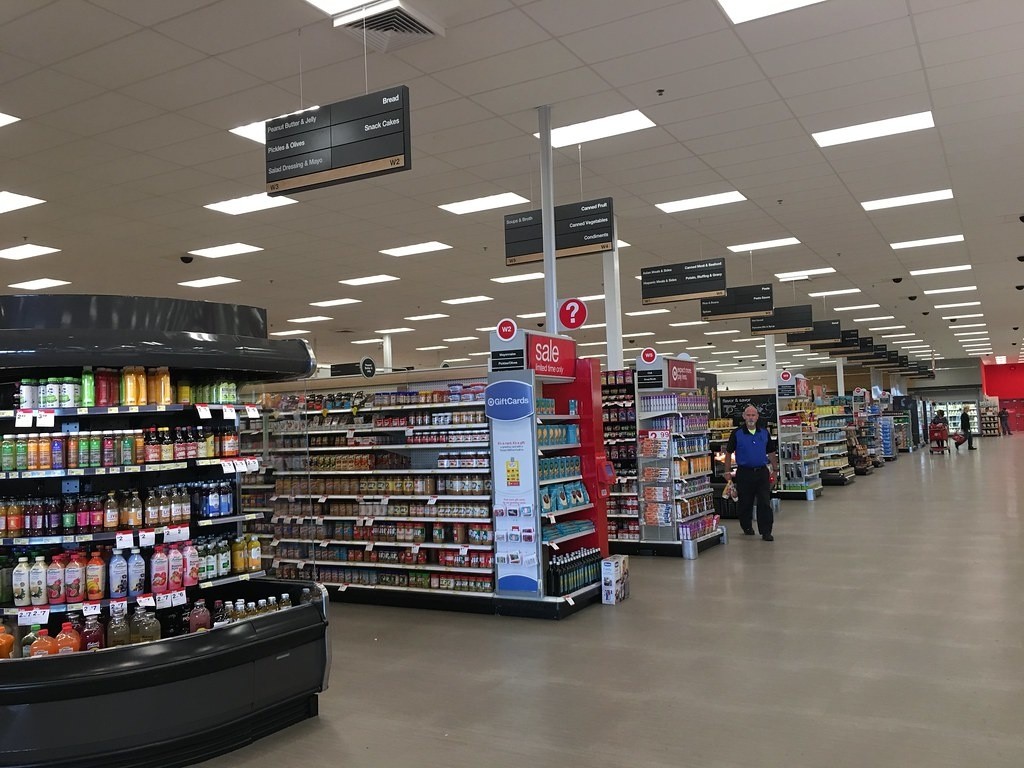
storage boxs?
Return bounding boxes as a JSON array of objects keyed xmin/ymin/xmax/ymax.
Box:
[{"xmin": 601, "ymin": 554, "xmax": 630, "ymax": 605}]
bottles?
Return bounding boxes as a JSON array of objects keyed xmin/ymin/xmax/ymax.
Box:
[
  {"xmin": 0, "ymin": 531, "xmax": 291, "ymax": 659},
  {"xmin": 600, "ymin": 369, "xmax": 641, "ymax": 540},
  {"xmin": 12, "ymin": 365, "xmax": 238, "ymax": 410},
  {"xmin": 722, "ymin": 480, "xmax": 739, "ymax": 502},
  {"xmin": 546, "ymin": 547, "xmax": 603, "ymax": 597},
  {"xmin": 0, "ymin": 478, "xmax": 235, "ymax": 538},
  {"xmin": 300, "ymin": 588, "xmax": 312, "ymax": 604},
  {"xmin": 934, "ymin": 404, "xmax": 978, "ymax": 433},
  {"xmin": 0, "ymin": 425, "xmax": 241, "ymax": 472}
]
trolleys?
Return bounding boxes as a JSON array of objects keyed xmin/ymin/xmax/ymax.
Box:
[{"xmin": 928, "ymin": 422, "xmax": 951, "ymax": 455}]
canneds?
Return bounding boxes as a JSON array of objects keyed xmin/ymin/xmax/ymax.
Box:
[
  {"xmin": 242, "ymin": 389, "xmax": 496, "ymax": 593},
  {"xmin": 602, "ymin": 407, "xmax": 636, "ymax": 459}
]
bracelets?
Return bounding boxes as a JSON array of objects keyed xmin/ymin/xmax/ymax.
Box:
[
  {"xmin": 773, "ymin": 467, "xmax": 777, "ymax": 471},
  {"xmin": 725, "ymin": 472, "xmax": 731, "ymax": 474}
]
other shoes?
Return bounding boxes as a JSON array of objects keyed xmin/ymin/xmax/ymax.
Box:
[
  {"xmin": 763, "ymin": 534, "xmax": 773, "ymax": 541},
  {"xmin": 968, "ymin": 447, "xmax": 977, "ymax": 450},
  {"xmin": 955, "ymin": 442, "xmax": 959, "ymax": 449},
  {"xmin": 741, "ymin": 524, "xmax": 755, "ymax": 534}
]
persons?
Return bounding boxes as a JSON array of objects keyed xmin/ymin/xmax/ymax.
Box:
[
  {"xmin": 955, "ymin": 406, "xmax": 977, "ymax": 450},
  {"xmin": 930, "ymin": 410, "xmax": 948, "ymax": 454},
  {"xmin": 724, "ymin": 405, "xmax": 778, "ymax": 541},
  {"xmin": 998, "ymin": 408, "xmax": 1013, "ymax": 435}
]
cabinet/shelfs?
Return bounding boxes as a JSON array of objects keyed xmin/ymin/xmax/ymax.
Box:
[
  {"xmin": 601, "ymin": 347, "xmax": 727, "ymax": 561},
  {"xmin": 237, "ymin": 318, "xmax": 610, "ymax": 621},
  {"xmin": 710, "ymin": 369, "xmax": 999, "ymax": 519},
  {"xmin": 0, "ymin": 295, "xmax": 330, "ymax": 768}
]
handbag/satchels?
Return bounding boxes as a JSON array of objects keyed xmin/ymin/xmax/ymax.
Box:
[{"xmin": 952, "ymin": 433, "xmax": 965, "ymax": 443}]
